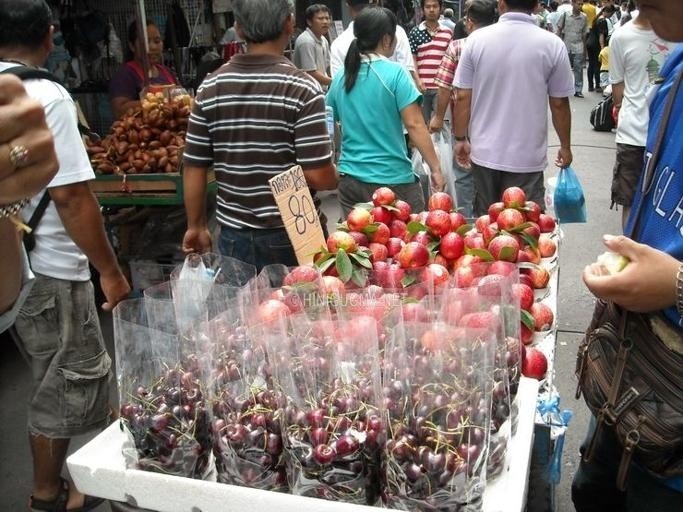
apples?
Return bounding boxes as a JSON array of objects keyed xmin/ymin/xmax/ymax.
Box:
[
  {"xmin": 596, "ymin": 250, "xmax": 628, "ymax": 277},
  {"xmin": 255, "ymin": 186, "xmax": 557, "ymax": 379}
]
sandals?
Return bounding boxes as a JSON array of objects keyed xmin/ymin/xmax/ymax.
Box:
[{"xmin": 29, "ymin": 476, "xmax": 104, "ymax": 511}]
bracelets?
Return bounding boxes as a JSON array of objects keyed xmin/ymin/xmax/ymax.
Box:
[
  {"xmin": 676, "ymin": 263, "xmax": 683, "ymax": 317},
  {"xmin": 0, "ymin": 199, "xmax": 31, "ymax": 220}
]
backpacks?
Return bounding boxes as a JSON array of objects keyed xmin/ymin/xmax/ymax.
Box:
[{"xmin": 590, "ymin": 93, "xmax": 612, "ymax": 131}]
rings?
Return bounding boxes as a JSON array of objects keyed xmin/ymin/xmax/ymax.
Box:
[{"xmin": 7, "ymin": 143, "xmax": 29, "ymax": 171}]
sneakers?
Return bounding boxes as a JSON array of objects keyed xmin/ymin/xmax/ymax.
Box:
[{"xmin": 574, "ymin": 87, "xmax": 602, "ymax": 97}]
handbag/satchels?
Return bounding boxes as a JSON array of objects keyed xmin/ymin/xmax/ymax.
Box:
[
  {"xmin": 575, "ymin": 300, "xmax": 683, "ymax": 480},
  {"xmin": 586, "ymin": 27, "xmax": 592, "ymax": 40}
]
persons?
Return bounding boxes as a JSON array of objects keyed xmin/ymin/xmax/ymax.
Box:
[
  {"xmin": 56, "ymin": 0, "xmax": 683, "ymax": 286},
  {"xmin": 0, "ymin": 73, "xmax": 59, "ymax": 335},
  {"xmin": 0, "ymin": 0, "xmax": 131, "ymax": 512},
  {"xmin": 571, "ymin": 1, "xmax": 683, "ymax": 511}
]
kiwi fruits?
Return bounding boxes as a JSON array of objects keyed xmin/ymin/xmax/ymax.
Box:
[{"xmin": 87, "ymin": 108, "xmax": 189, "ymax": 174}]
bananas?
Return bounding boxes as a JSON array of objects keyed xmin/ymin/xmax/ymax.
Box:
[{"xmin": 143, "ymin": 91, "xmax": 195, "ymax": 114}]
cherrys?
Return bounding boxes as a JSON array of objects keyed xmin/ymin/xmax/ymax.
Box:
[{"xmin": 119, "ymin": 322, "xmax": 518, "ymax": 512}]
